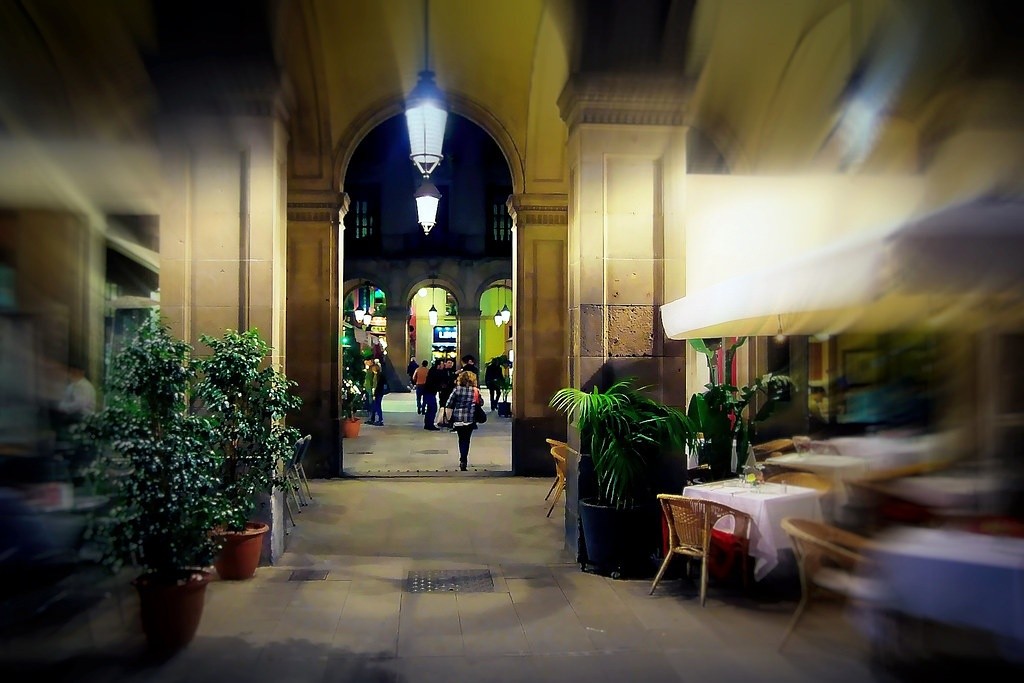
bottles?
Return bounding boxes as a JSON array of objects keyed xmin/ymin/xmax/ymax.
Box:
[{"xmin": 738, "ymin": 474, "xmax": 745, "ymax": 488}]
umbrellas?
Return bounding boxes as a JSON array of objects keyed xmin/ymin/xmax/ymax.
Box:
[{"xmin": 659, "ymin": 166, "xmax": 1024, "ymax": 462}]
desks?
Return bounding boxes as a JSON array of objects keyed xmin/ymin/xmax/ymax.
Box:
[
  {"xmin": 899, "ymin": 473, "xmax": 1022, "ymax": 517},
  {"xmin": 682, "ymin": 477, "xmax": 825, "ymax": 582},
  {"xmin": 810, "ymin": 436, "xmax": 966, "ymax": 468},
  {"xmin": 847, "ymin": 526, "xmax": 1024, "ymax": 674},
  {"xmin": 765, "ymin": 450, "xmax": 869, "ymax": 525}
]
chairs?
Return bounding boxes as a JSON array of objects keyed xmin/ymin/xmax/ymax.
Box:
[
  {"xmin": 284, "ymin": 433, "xmax": 313, "ymax": 536},
  {"xmin": 543, "ymin": 438, "xmax": 567, "ymax": 518},
  {"xmin": 647, "ymin": 437, "xmax": 1024, "ymax": 652}
]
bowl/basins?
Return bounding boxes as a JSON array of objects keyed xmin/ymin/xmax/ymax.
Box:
[{"xmin": 811, "ymin": 441, "xmax": 831, "ymax": 454}]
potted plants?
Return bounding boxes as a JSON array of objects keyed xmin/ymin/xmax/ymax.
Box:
[
  {"xmin": 68, "ymin": 307, "xmax": 232, "ymax": 650},
  {"xmin": 343, "ymin": 347, "xmax": 366, "ymax": 438},
  {"xmin": 187, "ymin": 326, "xmax": 303, "ymax": 581},
  {"xmin": 484, "ymin": 354, "xmax": 512, "ymax": 418},
  {"xmin": 548, "ymin": 375, "xmax": 699, "ymax": 581}
]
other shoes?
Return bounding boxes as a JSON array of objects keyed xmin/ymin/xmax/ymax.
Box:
[
  {"xmin": 373, "ymin": 421, "xmax": 383, "ymax": 426},
  {"xmin": 424, "ymin": 424, "xmax": 440, "ymax": 430},
  {"xmin": 460, "ymin": 463, "xmax": 466, "ymax": 471},
  {"xmin": 364, "ymin": 420, "xmax": 374, "ymax": 425}
]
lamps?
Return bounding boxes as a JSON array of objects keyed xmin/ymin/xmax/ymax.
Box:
[
  {"xmin": 494, "ymin": 279, "xmax": 511, "ymax": 328},
  {"xmin": 404, "ymin": 0, "xmax": 450, "ymax": 236},
  {"xmin": 354, "ymin": 278, "xmax": 372, "ymax": 327},
  {"xmin": 429, "ymin": 288, "xmax": 437, "ymax": 326}
]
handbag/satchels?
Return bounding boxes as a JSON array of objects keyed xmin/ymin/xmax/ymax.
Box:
[
  {"xmin": 437, "ymin": 408, "xmax": 454, "ymax": 428},
  {"xmin": 473, "ymin": 387, "xmax": 487, "ymax": 423}
]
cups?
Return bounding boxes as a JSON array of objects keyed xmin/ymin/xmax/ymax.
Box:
[
  {"xmin": 793, "ymin": 436, "xmax": 811, "ymax": 457},
  {"xmin": 781, "ymin": 480, "xmax": 787, "ymax": 494}
]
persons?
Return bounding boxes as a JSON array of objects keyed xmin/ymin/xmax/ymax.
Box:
[
  {"xmin": 413, "ymin": 359, "xmax": 429, "ymax": 415},
  {"xmin": 446, "ymin": 371, "xmax": 484, "ymax": 471},
  {"xmin": 55, "ymin": 354, "xmax": 96, "ymax": 425},
  {"xmin": 458, "ymin": 355, "xmax": 480, "ymax": 390},
  {"xmin": 809, "ymin": 386, "xmax": 826, "ymax": 422},
  {"xmin": 422, "ymin": 360, "xmax": 444, "ymax": 430},
  {"xmin": 485, "ymin": 358, "xmax": 505, "ymax": 411},
  {"xmin": 364, "ymin": 364, "xmax": 384, "ymax": 426},
  {"xmin": 437, "ymin": 358, "xmax": 459, "ymax": 427},
  {"xmin": 407, "ymin": 357, "xmax": 419, "ymax": 390}
]
